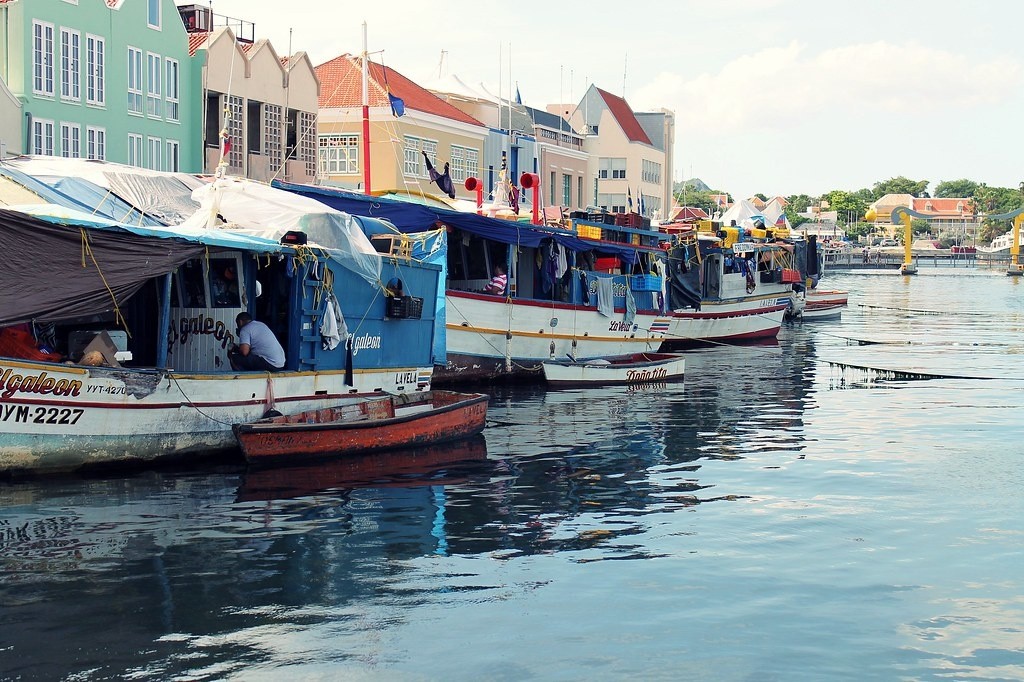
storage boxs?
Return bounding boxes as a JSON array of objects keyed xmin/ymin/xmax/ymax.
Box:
[
  {"xmin": 770, "ymin": 270, "xmax": 802, "ymax": 283},
  {"xmin": 72, "ymin": 329, "xmax": 119, "ymax": 367},
  {"xmin": 950, "ymin": 244, "xmax": 980, "ymax": 253},
  {"xmin": 569, "ymin": 220, "xmax": 602, "ymax": 240},
  {"xmin": 631, "ymin": 274, "xmax": 663, "ymax": 291},
  {"xmin": 385, "ymin": 295, "xmax": 423, "ymax": 319}
]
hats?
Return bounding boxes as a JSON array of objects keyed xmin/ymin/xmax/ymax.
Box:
[{"xmin": 876, "ymin": 244, "xmax": 879, "ymax": 246}]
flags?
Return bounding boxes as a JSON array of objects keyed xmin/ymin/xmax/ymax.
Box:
[{"xmin": 389, "ymin": 94, "xmax": 404, "ymax": 117}]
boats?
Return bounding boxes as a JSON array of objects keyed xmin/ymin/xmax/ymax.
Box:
[
  {"xmin": 1007, "ymin": 264, "xmax": 1024, "ymax": 276},
  {"xmin": 266, "ymin": 21, "xmax": 674, "ymax": 385},
  {"xmin": 798, "ymin": 290, "xmax": 848, "ymax": 304},
  {"xmin": 795, "ymin": 306, "xmax": 843, "ymax": 320},
  {"xmin": 900, "ymin": 263, "xmax": 918, "ymax": 274},
  {"xmin": 234, "ymin": 432, "xmax": 486, "ymax": 506},
  {"xmin": 232, "ymin": 390, "xmax": 489, "ymax": 462},
  {"xmin": 653, "ymin": 215, "xmax": 793, "ymax": 345},
  {"xmin": 0, "ymin": 151, "xmax": 452, "ymax": 470},
  {"xmin": 540, "ymin": 352, "xmax": 685, "ymax": 385}
]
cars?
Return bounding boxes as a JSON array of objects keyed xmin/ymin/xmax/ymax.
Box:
[
  {"xmin": 872, "ymin": 237, "xmax": 891, "ymax": 246},
  {"xmin": 850, "ymin": 240, "xmax": 866, "ymax": 248},
  {"xmin": 881, "ymin": 239, "xmax": 898, "ymax": 247}
]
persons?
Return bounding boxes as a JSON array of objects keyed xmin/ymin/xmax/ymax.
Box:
[
  {"xmin": 875, "ymin": 247, "xmax": 881, "ymax": 265},
  {"xmin": 226, "ymin": 312, "xmax": 286, "ymax": 371},
  {"xmin": 479, "ymin": 263, "xmax": 507, "ymax": 296},
  {"xmin": 863, "ymin": 246, "xmax": 871, "ymax": 264}
]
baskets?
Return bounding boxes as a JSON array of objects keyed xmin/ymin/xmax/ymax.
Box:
[{"xmin": 386, "ymin": 293, "xmax": 424, "ymax": 320}]
